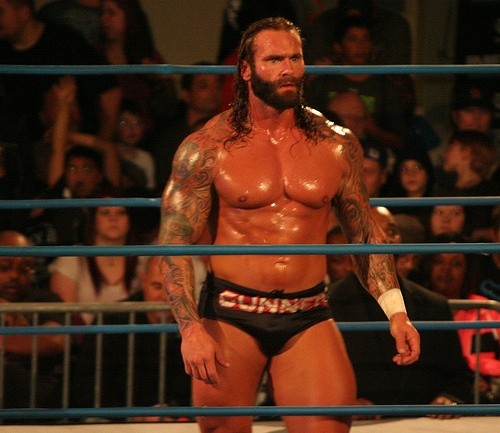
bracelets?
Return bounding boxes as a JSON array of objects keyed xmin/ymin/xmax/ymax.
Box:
[{"xmin": 376, "ymin": 288, "xmax": 407, "ymax": 319}]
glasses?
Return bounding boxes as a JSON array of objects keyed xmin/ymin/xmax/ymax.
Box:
[
  {"xmin": 66, "ymin": 165, "xmax": 97, "ymax": 175},
  {"xmin": 119, "ymin": 120, "xmax": 142, "ymax": 127}
]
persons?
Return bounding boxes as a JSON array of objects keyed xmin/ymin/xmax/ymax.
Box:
[
  {"xmin": 46, "ymin": 194, "xmax": 151, "ymax": 342},
  {"xmin": 69, "ymin": 236, "xmax": 207, "ymax": 420},
  {"xmin": 252, "ymin": 206, "xmax": 472, "ymax": 421},
  {"xmin": 156, "ymin": 17, "xmax": 420, "ymax": 433},
  {"xmin": 0, "ymin": 230, "xmax": 78, "ymax": 409},
  {"xmin": 0, "ymin": 0, "xmax": 500, "ymax": 401}
]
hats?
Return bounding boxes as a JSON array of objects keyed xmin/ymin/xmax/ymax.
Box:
[{"xmin": 360, "ymin": 138, "xmax": 388, "ymax": 170}]
